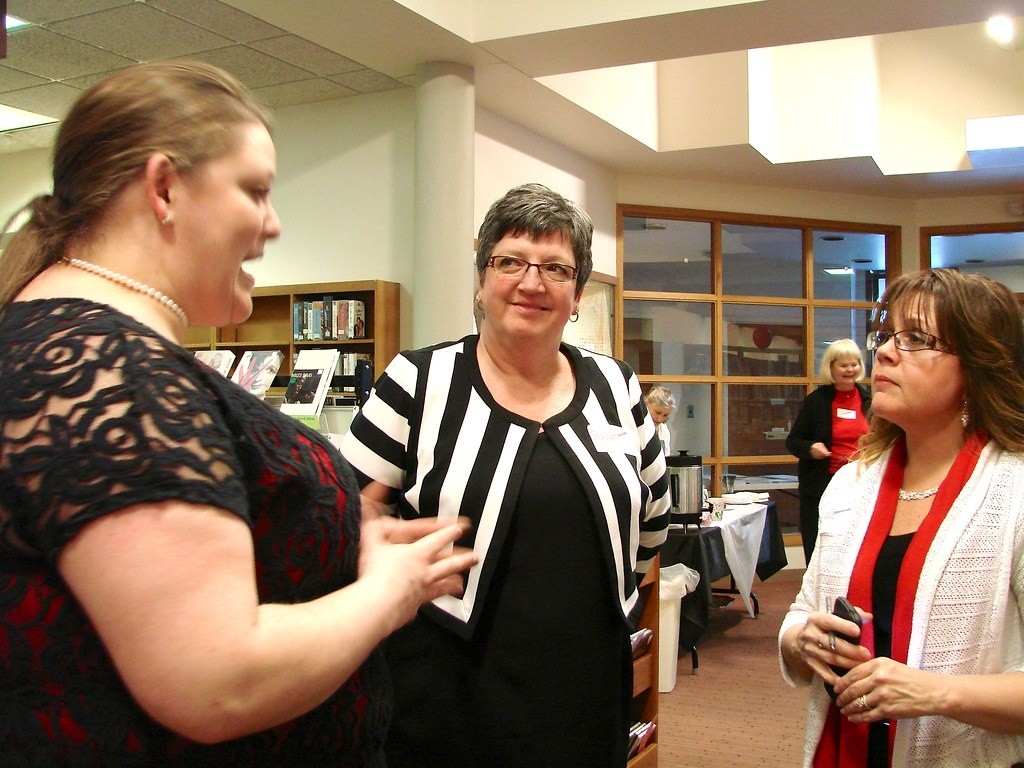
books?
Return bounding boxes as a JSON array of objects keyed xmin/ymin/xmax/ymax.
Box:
[
  {"xmin": 292, "ymin": 295, "xmax": 365, "ymax": 341},
  {"xmin": 193, "ymin": 350, "xmax": 236, "ymax": 379},
  {"xmin": 626, "ymin": 714, "xmax": 658, "ymax": 761},
  {"xmin": 629, "ymin": 627, "xmax": 654, "ymax": 662},
  {"xmin": 293, "ymin": 353, "xmax": 371, "ymax": 393},
  {"xmin": 229, "ymin": 350, "xmax": 286, "ymax": 402},
  {"xmin": 279, "ymin": 349, "xmax": 340, "ymax": 419}
]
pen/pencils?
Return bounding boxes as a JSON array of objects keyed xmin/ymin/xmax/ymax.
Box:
[{"xmin": 824, "ymin": 596, "xmax": 836, "ymax": 652}]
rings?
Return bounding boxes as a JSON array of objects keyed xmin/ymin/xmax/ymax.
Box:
[
  {"xmin": 818, "ymin": 632, "xmax": 826, "ymax": 648},
  {"xmin": 859, "ymin": 694, "xmax": 873, "ymax": 711}
]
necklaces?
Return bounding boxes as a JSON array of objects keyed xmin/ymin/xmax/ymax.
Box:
[
  {"xmin": 898, "ymin": 485, "xmax": 941, "ymax": 502},
  {"xmin": 54, "ymin": 250, "xmax": 192, "ymax": 325}
]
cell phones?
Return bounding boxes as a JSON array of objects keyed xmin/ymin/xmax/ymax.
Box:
[{"xmin": 824, "ymin": 597, "xmax": 863, "ymax": 702}]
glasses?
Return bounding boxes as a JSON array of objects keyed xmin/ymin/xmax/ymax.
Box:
[
  {"xmin": 866, "ymin": 329, "xmax": 958, "ymax": 356},
  {"xmin": 487, "ymin": 256, "xmax": 578, "ymax": 284}
]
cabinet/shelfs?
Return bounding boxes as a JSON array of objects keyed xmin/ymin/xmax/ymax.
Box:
[
  {"xmin": 180, "ymin": 278, "xmax": 401, "ymax": 408},
  {"xmin": 627, "ymin": 555, "xmax": 659, "ymax": 768}
]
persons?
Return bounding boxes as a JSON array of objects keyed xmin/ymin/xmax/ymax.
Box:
[
  {"xmin": 643, "ymin": 386, "xmax": 677, "ymax": 457},
  {"xmin": 784, "ymin": 337, "xmax": 873, "ymax": 574},
  {"xmin": 342, "ymin": 182, "xmax": 674, "ymax": 768},
  {"xmin": 0, "ymin": 59, "xmax": 481, "ymax": 768},
  {"xmin": 774, "ymin": 266, "xmax": 1024, "ymax": 768}
]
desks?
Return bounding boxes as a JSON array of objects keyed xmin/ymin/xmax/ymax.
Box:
[
  {"xmin": 701, "ymin": 474, "xmax": 800, "ymax": 506},
  {"xmin": 660, "ymin": 496, "xmax": 787, "ymax": 677}
]
